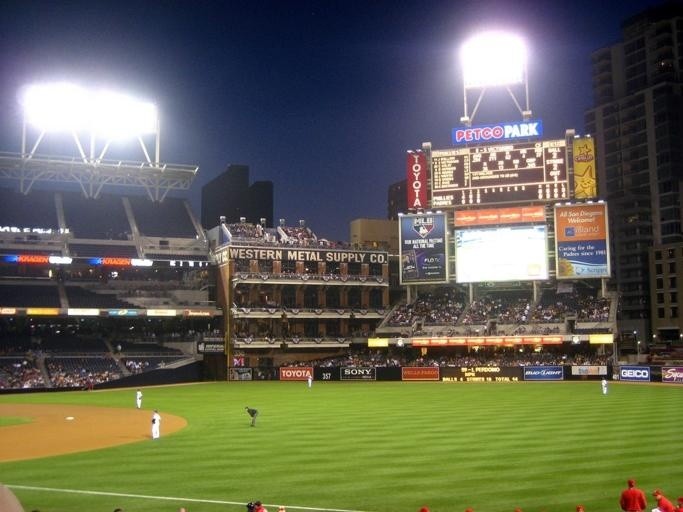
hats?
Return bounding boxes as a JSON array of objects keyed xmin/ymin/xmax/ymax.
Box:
[
  {"xmin": 576, "ymin": 505, "xmax": 584, "ymax": 511},
  {"xmin": 652, "ymin": 489, "xmax": 662, "ymax": 496},
  {"xmin": 677, "ymin": 496, "xmax": 683, "ymax": 503},
  {"xmin": 421, "ymin": 506, "xmax": 521, "ymax": 512},
  {"xmin": 628, "ymin": 479, "xmax": 635, "ymax": 486},
  {"xmin": 247, "ymin": 500, "xmax": 261, "ymax": 510}
]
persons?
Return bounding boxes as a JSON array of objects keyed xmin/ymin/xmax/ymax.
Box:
[
  {"xmin": 0, "ymin": 359, "xmax": 150, "ymax": 388},
  {"xmin": 226, "ymin": 224, "xmax": 316, "ymax": 247},
  {"xmin": 404, "ymin": 255, "xmax": 414, "ymax": 269},
  {"xmin": 65, "ymin": 267, "xmax": 166, "ymax": 281},
  {"xmin": 239, "ymin": 301, "xmax": 327, "ymax": 309},
  {"xmin": 0, "ymin": 486, "xmax": 24, "ymax": 512},
  {"xmin": 234, "ymin": 331, "xmax": 306, "ymax": 337},
  {"xmin": 651, "ymin": 489, "xmax": 683, "ymax": 512},
  {"xmin": 307, "ymin": 373, "xmax": 313, "ymax": 387},
  {"xmin": 136, "ymin": 388, "xmax": 143, "ymax": 410},
  {"xmin": 245, "ymin": 407, "xmax": 257, "ymax": 426},
  {"xmin": 157, "ymin": 360, "xmax": 166, "ymax": 368},
  {"xmin": 282, "ymin": 347, "xmax": 614, "ymax": 368},
  {"xmin": 329, "ymin": 240, "xmax": 386, "ymax": 251},
  {"xmin": 601, "ymin": 377, "xmax": 608, "ymax": 395},
  {"xmin": 387, "ymin": 289, "xmax": 611, "ymax": 338},
  {"xmin": 88, "ymin": 382, "xmax": 94, "ymax": 392},
  {"xmin": 144, "ymin": 328, "xmax": 208, "ymax": 339},
  {"xmin": 82, "ymin": 383, "xmax": 88, "ymax": 391},
  {"xmin": 620, "ymin": 478, "xmax": 648, "ymax": 512},
  {"xmin": 255, "ymin": 499, "xmax": 267, "ymax": 512},
  {"xmin": 152, "ymin": 411, "xmax": 161, "ymax": 440},
  {"xmin": 283, "ymin": 270, "xmax": 351, "ymax": 280},
  {"xmin": 352, "ymin": 327, "xmax": 373, "ymax": 337}
]
